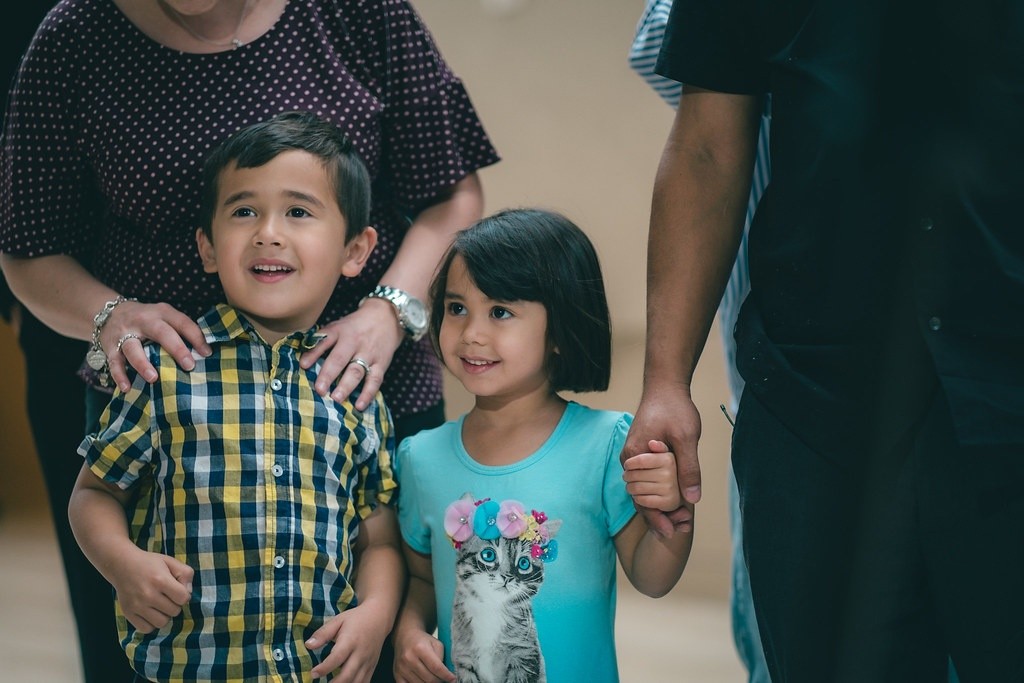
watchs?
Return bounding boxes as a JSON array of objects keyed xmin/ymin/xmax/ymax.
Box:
[{"xmin": 359, "ymin": 282, "xmax": 432, "ymax": 343}]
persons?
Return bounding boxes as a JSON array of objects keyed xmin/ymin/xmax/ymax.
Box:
[
  {"xmin": 1, "ymin": 0, "xmax": 503, "ymax": 682},
  {"xmin": 620, "ymin": 0, "xmax": 1024, "ymax": 683},
  {"xmin": 390, "ymin": 209, "xmax": 695, "ymax": 683},
  {"xmin": 70, "ymin": 108, "xmax": 410, "ymax": 680}
]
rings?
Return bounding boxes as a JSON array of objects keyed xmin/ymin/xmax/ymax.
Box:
[
  {"xmin": 351, "ymin": 358, "xmax": 370, "ymax": 374},
  {"xmin": 115, "ymin": 334, "xmax": 141, "ymax": 351}
]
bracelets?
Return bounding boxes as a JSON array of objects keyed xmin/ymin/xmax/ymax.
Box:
[{"xmin": 87, "ymin": 294, "xmax": 138, "ymax": 388}]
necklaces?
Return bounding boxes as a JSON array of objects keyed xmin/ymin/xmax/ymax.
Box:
[{"xmin": 168, "ymin": 4, "xmax": 246, "ymax": 49}]
